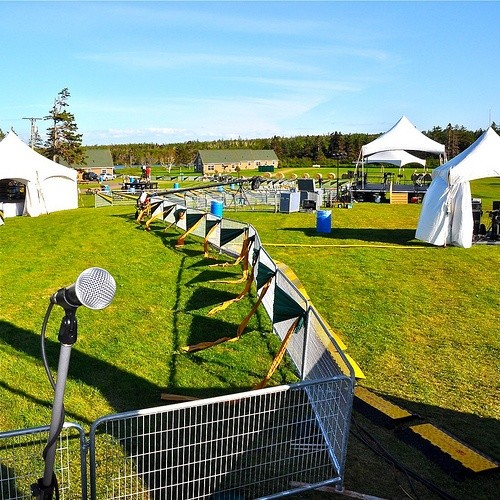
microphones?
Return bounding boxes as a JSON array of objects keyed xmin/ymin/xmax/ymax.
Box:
[{"xmin": 50, "ymin": 267, "xmax": 117, "ymax": 310}]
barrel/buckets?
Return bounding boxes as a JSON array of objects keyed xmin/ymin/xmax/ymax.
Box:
[
  {"xmin": 210, "ymin": 201, "xmax": 223, "ymax": 219},
  {"xmin": 217, "ymin": 186, "xmax": 223, "ymax": 192},
  {"xmin": 173, "ymin": 183, "xmax": 180, "ymax": 190},
  {"xmin": 230, "ymin": 184, "xmax": 234, "ymax": 190},
  {"xmin": 316, "ymin": 210, "xmax": 331, "ymax": 233}
]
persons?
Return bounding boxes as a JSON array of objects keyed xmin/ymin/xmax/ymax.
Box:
[{"xmin": 141, "ymin": 166, "xmax": 151, "ymax": 183}]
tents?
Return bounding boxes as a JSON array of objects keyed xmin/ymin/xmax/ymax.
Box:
[
  {"xmin": 0, "ymin": 129, "xmax": 78, "ymax": 219},
  {"xmin": 414, "ymin": 127, "xmax": 500, "ymax": 248},
  {"xmin": 352, "ymin": 115, "xmax": 447, "ymax": 190}
]
